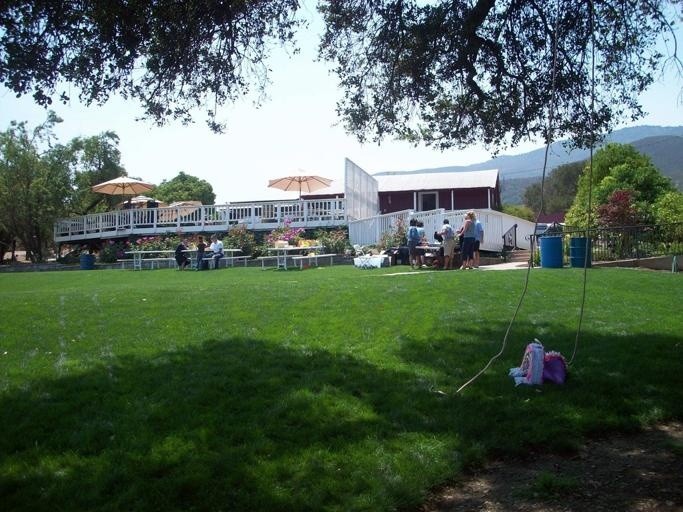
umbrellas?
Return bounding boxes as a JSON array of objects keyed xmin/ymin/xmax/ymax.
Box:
[
  {"xmin": 114, "ymin": 195, "xmax": 167, "ymax": 209},
  {"xmin": 158, "ymin": 200, "xmax": 203, "ymax": 221},
  {"xmin": 92, "ymin": 174, "xmax": 153, "ymax": 209},
  {"xmin": 267, "ymin": 176, "xmax": 333, "ymax": 201}
]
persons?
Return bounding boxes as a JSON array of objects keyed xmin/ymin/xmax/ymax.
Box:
[
  {"xmin": 123, "ymin": 198, "xmax": 134, "ymax": 209},
  {"xmin": 406, "ymin": 211, "xmax": 484, "ymax": 269},
  {"xmin": 175, "ymin": 239, "xmax": 191, "ymax": 271},
  {"xmin": 196, "ymin": 236, "xmax": 206, "ymax": 270},
  {"xmin": 203, "ymin": 235, "xmax": 224, "ymax": 269}
]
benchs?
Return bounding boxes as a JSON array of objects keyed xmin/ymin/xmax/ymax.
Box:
[
  {"xmin": 256, "ymin": 255, "xmax": 302, "ymax": 270},
  {"xmin": 291, "ymin": 254, "xmax": 335, "ymax": 270},
  {"xmin": 117, "ymin": 256, "xmax": 251, "ymax": 269}
]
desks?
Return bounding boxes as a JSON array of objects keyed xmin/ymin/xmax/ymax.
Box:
[
  {"xmin": 267, "ymin": 248, "xmax": 317, "ymax": 270},
  {"xmin": 125, "ymin": 249, "xmax": 242, "ymax": 270},
  {"xmin": 415, "ymin": 243, "xmax": 462, "ymax": 269}
]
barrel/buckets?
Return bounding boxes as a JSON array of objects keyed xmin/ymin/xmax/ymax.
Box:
[
  {"xmin": 540, "ymin": 236, "xmax": 563, "ymax": 268},
  {"xmin": 569, "ymin": 237, "xmax": 592, "ymax": 268},
  {"xmin": 80, "ymin": 255, "xmax": 97, "ymax": 270}
]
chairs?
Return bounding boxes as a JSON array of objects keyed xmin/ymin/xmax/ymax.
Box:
[{"xmin": 353, "ymin": 244, "xmax": 370, "ymax": 269}]
